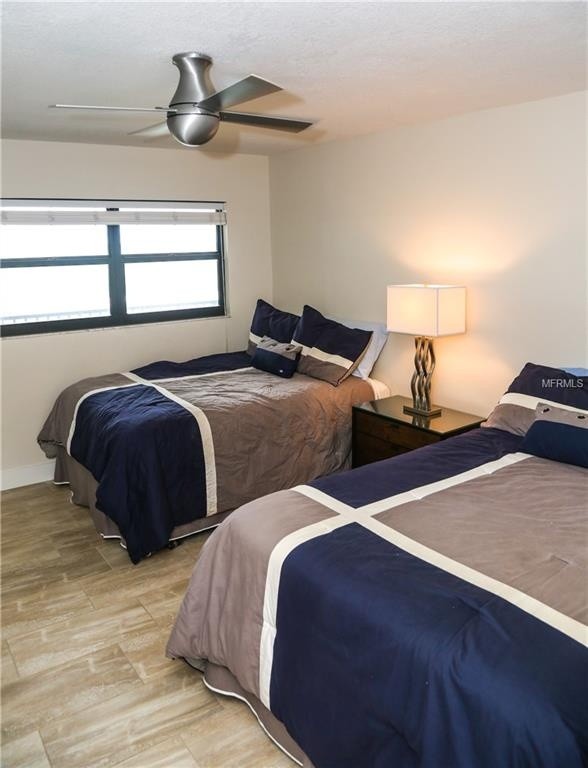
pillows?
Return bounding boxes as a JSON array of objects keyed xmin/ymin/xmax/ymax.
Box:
[
  {"xmin": 516, "ymin": 401, "xmax": 588, "ymax": 469},
  {"xmin": 249, "ymin": 334, "xmax": 304, "ymax": 380},
  {"xmin": 246, "ymin": 297, "xmax": 302, "ymax": 357},
  {"xmin": 288, "ymin": 304, "xmax": 374, "ymax": 387},
  {"xmin": 478, "ymin": 362, "xmax": 588, "ymax": 438},
  {"xmin": 324, "ymin": 315, "xmax": 391, "ymax": 380}
]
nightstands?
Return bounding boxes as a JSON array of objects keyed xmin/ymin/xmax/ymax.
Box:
[{"xmin": 351, "ymin": 394, "xmax": 486, "ymax": 469}]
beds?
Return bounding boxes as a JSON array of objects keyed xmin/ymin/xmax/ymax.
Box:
[
  {"xmin": 166, "ymin": 359, "xmax": 588, "ymax": 768},
  {"xmin": 33, "ymin": 296, "xmax": 392, "ymax": 568}
]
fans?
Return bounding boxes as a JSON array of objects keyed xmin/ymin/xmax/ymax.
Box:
[{"xmin": 46, "ymin": 50, "xmax": 314, "ymax": 148}]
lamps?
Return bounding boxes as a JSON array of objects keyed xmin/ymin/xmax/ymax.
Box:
[{"xmin": 385, "ymin": 282, "xmax": 467, "ymax": 417}]
